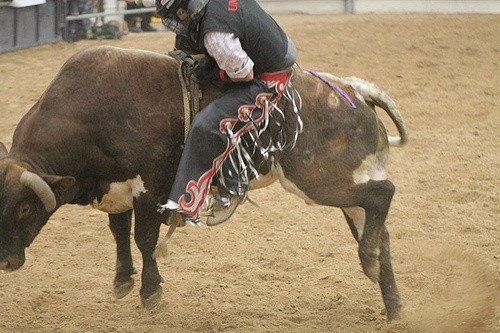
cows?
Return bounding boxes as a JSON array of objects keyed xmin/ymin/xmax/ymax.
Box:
[{"xmin": 0, "ymin": 45, "xmax": 408, "ymax": 321}]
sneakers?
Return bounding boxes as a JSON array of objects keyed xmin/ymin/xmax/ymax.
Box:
[{"xmin": 206, "ymin": 187, "xmax": 248, "ymax": 226}]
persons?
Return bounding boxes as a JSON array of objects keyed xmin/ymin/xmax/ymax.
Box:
[{"xmin": 158, "ymin": 0, "xmax": 297, "ymax": 227}]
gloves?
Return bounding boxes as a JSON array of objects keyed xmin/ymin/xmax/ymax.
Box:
[{"xmin": 189, "ymin": 56, "xmax": 228, "ymax": 85}]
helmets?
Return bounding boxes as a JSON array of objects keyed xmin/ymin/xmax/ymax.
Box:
[{"xmin": 155, "ymin": 0, "xmax": 189, "ymax": 21}]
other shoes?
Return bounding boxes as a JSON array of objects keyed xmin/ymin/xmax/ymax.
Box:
[
  {"xmin": 141, "ymin": 25, "xmax": 157, "ymax": 32},
  {"xmin": 129, "ymin": 25, "xmax": 140, "ymax": 32}
]
